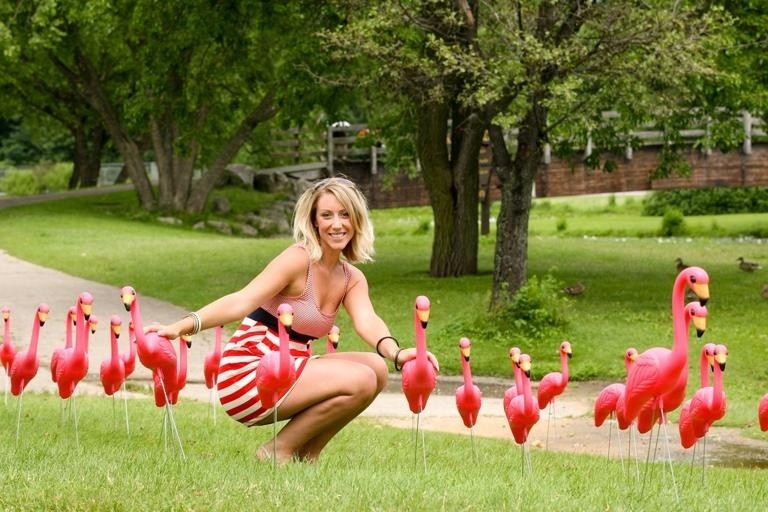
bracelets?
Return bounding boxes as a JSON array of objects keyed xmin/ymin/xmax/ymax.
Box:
[
  {"xmin": 394, "ymin": 347, "xmax": 406, "ymax": 372},
  {"xmin": 376, "ymin": 336, "xmax": 400, "ymax": 358},
  {"xmin": 181, "ymin": 312, "xmax": 202, "ymax": 336}
]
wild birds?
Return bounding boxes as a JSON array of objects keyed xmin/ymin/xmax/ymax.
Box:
[
  {"xmin": 398, "ymin": 294, "xmax": 440, "ymax": 474},
  {"xmin": 592, "ymin": 265, "xmax": 732, "ymax": 498},
  {"xmin": 452, "ymin": 336, "xmax": 486, "ymax": 464},
  {"xmin": 0, "ymin": 277, "xmax": 191, "ymax": 458},
  {"xmin": 323, "ymin": 322, "xmax": 342, "ymax": 354},
  {"xmin": 500, "ymin": 342, "xmax": 574, "ymax": 494},
  {"xmin": 253, "ymin": 302, "xmax": 299, "ymax": 467},
  {"xmin": 202, "ymin": 324, "xmax": 225, "ymax": 428}
]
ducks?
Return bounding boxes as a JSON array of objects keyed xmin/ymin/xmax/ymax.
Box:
[
  {"xmin": 565, "ymin": 278, "xmax": 587, "ymax": 297},
  {"xmin": 674, "ymin": 256, "xmax": 686, "ymax": 272},
  {"xmin": 734, "ymin": 255, "xmax": 761, "ymax": 275}
]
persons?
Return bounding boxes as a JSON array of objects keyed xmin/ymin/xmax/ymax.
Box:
[
  {"xmin": 131, "ymin": 176, "xmax": 442, "ymax": 469},
  {"xmin": 333, "ymin": 125, "xmax": 346, "ymax": 148}
]
